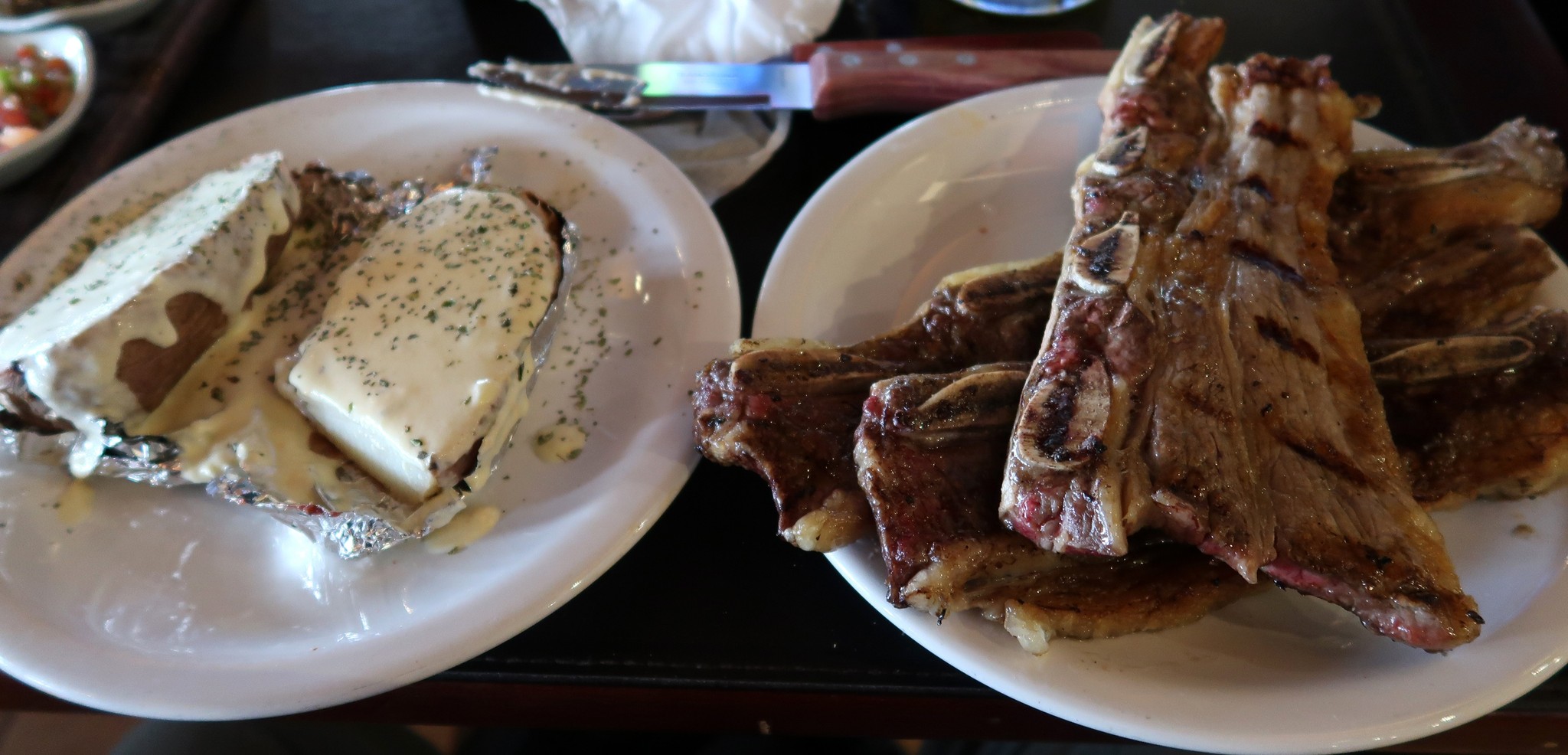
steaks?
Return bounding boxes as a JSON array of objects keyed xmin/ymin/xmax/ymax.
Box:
[{"xmin": 686, "ymin": 1, "xmax": 1566, "ymax": 656}]
